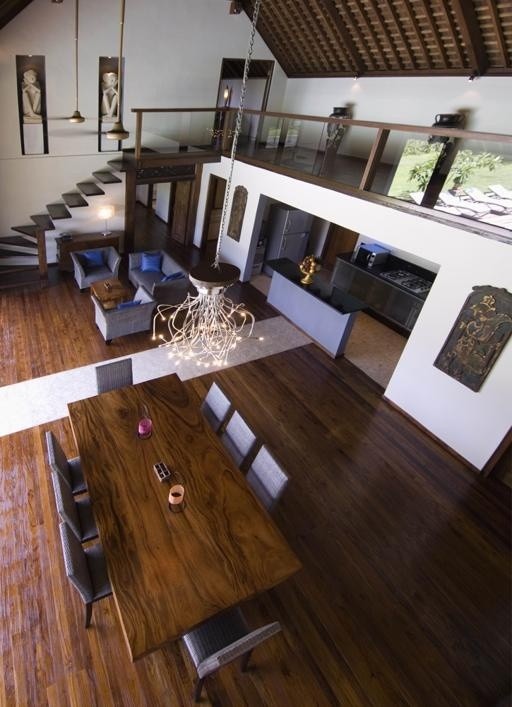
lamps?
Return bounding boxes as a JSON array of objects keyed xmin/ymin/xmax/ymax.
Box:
[
  {"xmin": 96, "ymin": 205, "xmax": 115, "ymax": 235},
  {"xmin": 222, "ymin": 85, "xmax": 230, "ymax": 107},
  {"xmin": 105, "ymin": 0, "xmax": 129, "ymax": 140},
  {"xmin": 69, "ymin": 0, "xmax": 85, "ymax": 123},
  {"xmin": 151, "ymin": 0, "xmax": 263, "ymax": 368}
]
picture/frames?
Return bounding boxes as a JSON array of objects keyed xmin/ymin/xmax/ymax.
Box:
[{"xmin": 433, "ymin": 286, "xmax": 512, "ymax": 393}]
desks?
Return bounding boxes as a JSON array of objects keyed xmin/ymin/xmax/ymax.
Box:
[
  {"xmin": 264, "ymin": 257, "xmax": 371, "ymax": 359},
  {"xmin": 66, "ymin": 372, "xmax": 302, "ymax": 664}
]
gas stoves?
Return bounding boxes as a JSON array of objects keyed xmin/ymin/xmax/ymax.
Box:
[{"xmin": 379, "ymin": 270, "xmax": 433, "ymax": 294}]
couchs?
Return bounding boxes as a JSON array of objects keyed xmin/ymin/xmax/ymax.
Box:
[
  {"xmin": 91, "ymin": 285, "xmax": 158, "ymax": 345},
  {"xmin": 69, "ymin": 246, "xmax": 121, "ymax": 294},
  {"xmin": 129, "ymin": 249, "xmax": 191, "ymax": 314}
]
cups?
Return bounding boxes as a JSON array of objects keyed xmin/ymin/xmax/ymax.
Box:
[
  {"xmin": 138, "ymin": 418, "xmax": 152, "ymax": 435},
  {"xmin": 169, "ymin": 485, "xmax": 184, "ymax": 504}
]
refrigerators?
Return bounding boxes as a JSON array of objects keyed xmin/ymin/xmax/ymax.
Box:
[{"xmin": 262, "ymin": 203, "xmax": 314, "ymax": 277}]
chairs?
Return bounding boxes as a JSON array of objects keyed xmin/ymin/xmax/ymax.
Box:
[
  {"xmin": 199, "ymin": 382, "xmax": 232, "ymax": 431},
  {"xmin": 243, "ymin": 446, "xmax": 290, "ymax": 513},
  {"xmin": 52, "ymin": 472, "xmax": 99, "ymax": 544},
  {"xmin": 58, "ymin": 523, "xmax": 113, "ymax": 629},
  {"xmin": 440, "ymin": 190, "xmax": 490, "ymax": 216},
  {"xmin": 95, "ymin": 358, "xmax": 132, "ymax": 395},
  {"xmin": 47, "ymin": 432, "xmax": 95, "ymax": 496},
  {"xmin": 411, "ymin": 190, "xmax": 478, "ymax": 221},
  {"xmin": 218, "ymin": 412, "xmax": 258, "ymax": 469},
  {"xmin": 464, "ymin": 188, "xmax": 512, "ymax": 213},
  {"xmin": 183, "ymin": 607, "xmax": 282, "ymax": 703},
  {"xmin": 489, "ymin": 185, "xmax": 512, "ymax": 200}
]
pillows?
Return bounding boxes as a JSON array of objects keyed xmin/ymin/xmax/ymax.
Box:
[
  {"xmin": 118, "ymin": 299, "xmax": 141, "ymax": 310},
  {"xmin": 140, "ymin": 253, "xmax": 160, "ymax": 270},
  {"xmin": 141, "ymin": 255, "xmax": 162, "ymax": 273},
  {"xmin": 161, "ymin": 271, "xmax": 181, "ymax": 281},
  {"xmin": 84, "ymin": 250, "xmax": 104, "ymax": 269}
]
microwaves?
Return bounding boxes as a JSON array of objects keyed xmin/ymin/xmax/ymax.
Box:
[{"xmin": 352, "ymin": 243, "xmax": 390, "ymax": 269}]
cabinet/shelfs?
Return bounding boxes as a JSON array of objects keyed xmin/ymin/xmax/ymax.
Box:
[
  {"xmin": 54, "ymin": 232, "xmax": 121, "ymax": 276},
  {"xmin": 329, "ymin": 257, "xmax": 424, "ymax": 339}
]
